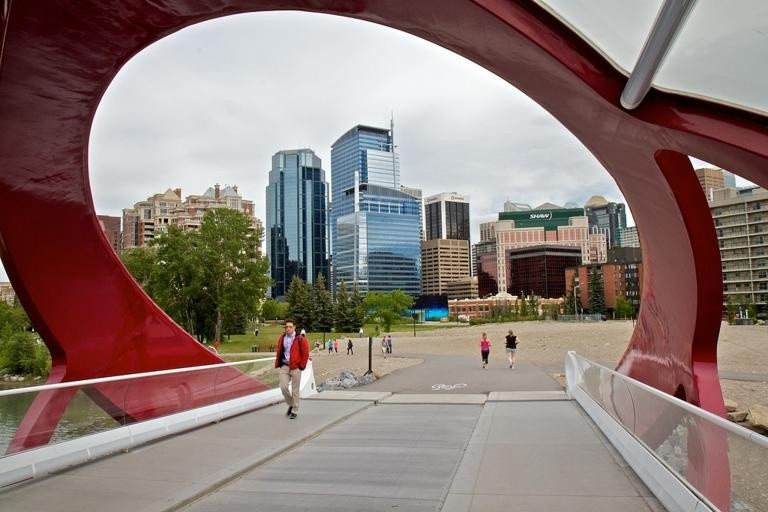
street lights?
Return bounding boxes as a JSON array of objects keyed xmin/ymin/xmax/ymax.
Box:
[{"xmin": 573, "ymin": 286, "xmax": 580, "ymax": 316}]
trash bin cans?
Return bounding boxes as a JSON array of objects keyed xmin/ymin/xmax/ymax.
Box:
[{"xmin": 252, "ymin": 347, "xmax": 257, "ymax": 352}]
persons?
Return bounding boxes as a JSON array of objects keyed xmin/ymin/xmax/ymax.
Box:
[
  {"xmin": 381, "ymin": 335, "xmax": 389, "ymax": 359},
  {"xmin": 479, "ymin": 332, "xmax": 491, "ymax": 370},
  {"xmin": 347, "ymin": 339, "xmax": 353, "ymax": 355},
  {"xmin": 504, "ymin": 329, "xmax": 521, "ymax": 370},
  {"xmin": 358, "ymin": 327, "xmax": 364, "ymax": 337},
  {"xmin": 328, "ymin": 338, "xmax": 334, "ymax": 356},
  {"xmin": 314, "ymin": 338, "xmax": 322, "ymax": 358},
  {"xmin": 274, "ymin": 317, "xmax": 309, "ymax": 418},
  {"xmin": 387, "ymin": 335, "xmax": 392, "ymax": 354},
  {"xmin": 333, "ymin": 338, "xmax": 338, "ymax": 353},
  {"xmin": 300, "ymin": 327, "xmax": 307, "ymax": 337}
]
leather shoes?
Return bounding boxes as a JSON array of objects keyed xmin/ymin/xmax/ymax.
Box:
[{"xmin": 287, "ymin": 406, "xmax": 298, "ymax": 419}]
